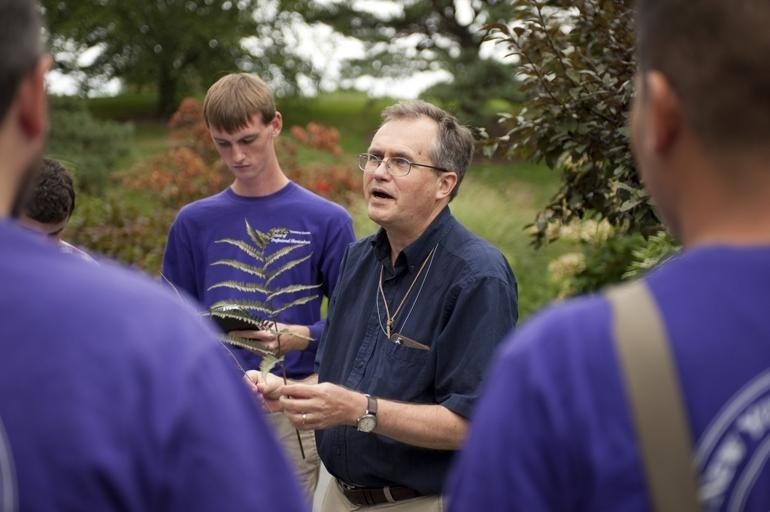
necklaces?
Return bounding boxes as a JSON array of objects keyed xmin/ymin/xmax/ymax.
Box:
[
  {"xmin": 374, "ymin": 243, "xmax": 439, "ymax": 339},
  {"xmin": 371, "ymin": 244, "xmax": 440, "ymax": 352}
]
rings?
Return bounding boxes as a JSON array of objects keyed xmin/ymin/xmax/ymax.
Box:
[{"xmin": 300, "ymin": 413, "xmax": 307, "ymax": 427}]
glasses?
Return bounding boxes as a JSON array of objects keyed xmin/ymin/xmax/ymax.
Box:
[{"xmin": 359, "ymin": 153, "xmax": 448, "ymax": 176}]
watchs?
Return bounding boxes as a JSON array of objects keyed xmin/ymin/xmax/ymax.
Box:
[{"xmin": 351, "ymin": 393, "xmax": 380, "ymax": 435}]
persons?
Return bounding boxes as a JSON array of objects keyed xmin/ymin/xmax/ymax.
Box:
[
  {"xmin": 436, "ymin": 1, "xmax": 770, "ymax": 511},
  {"xmin": 238, "ymin": 93, "xmax": 524, "ymax": 511},
  {"xmin": 5, "ymin": 154, "xmax": 104, "ymax": 272},
  {"xmin": 157, "ymin": 68, "xmax": 360, "ymax": 510},
  {"xmin": 0, "ymin": 0, "xmax": 315, "ymax": 512}
]
